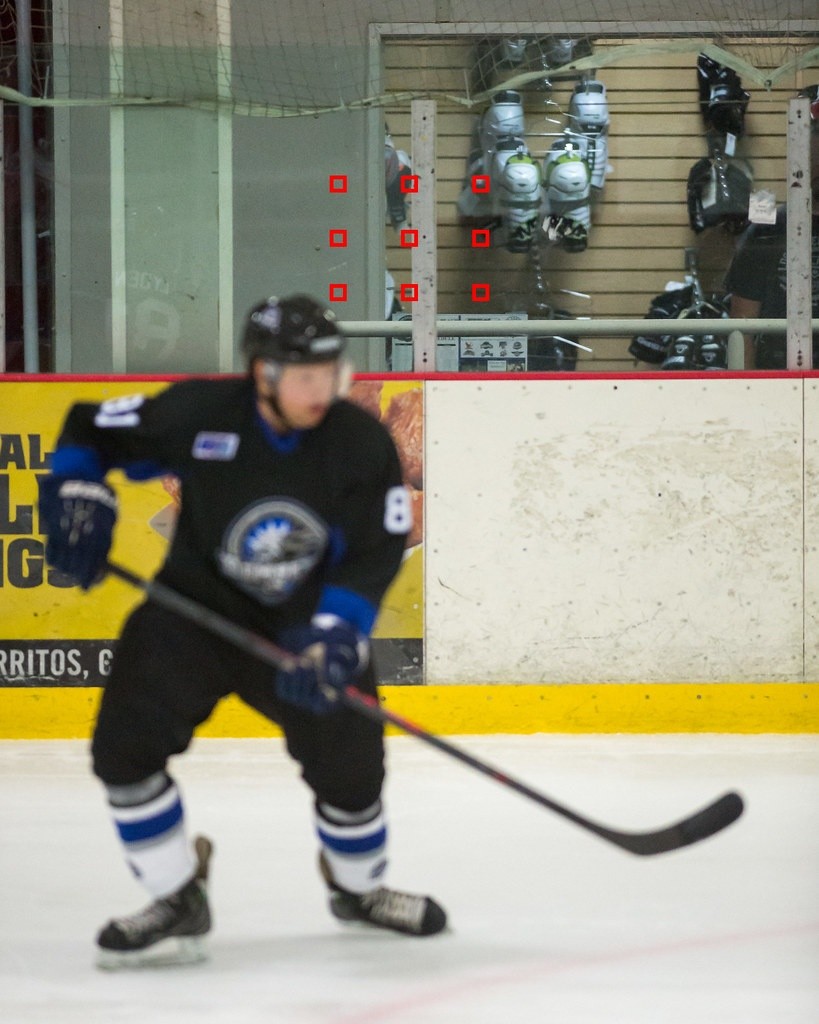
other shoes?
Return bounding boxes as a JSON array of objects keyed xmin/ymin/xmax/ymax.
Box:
[{"xmin": 662, "ymin": 298, "xmax": 729, "ymax": 372}]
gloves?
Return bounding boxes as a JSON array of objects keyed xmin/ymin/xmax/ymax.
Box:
[
  {"xmin": 48, "ymin": 477, "xmax": 118, "ymax": 589},
  {"xmin": 631, "ymin": 285, "xmax": 696, "ymax": 364},
  {"xmin": 697, "ymin": 52, "xmax": 749, "ymax": 139},
  {"xmin": 276, "ymin": 623, "xmax": 360, "ymax": 716}
]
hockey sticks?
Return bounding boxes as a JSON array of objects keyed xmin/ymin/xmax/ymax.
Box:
[{"xmin": 102, "ymin": 561, "xmax": 746, "ymax": 857}]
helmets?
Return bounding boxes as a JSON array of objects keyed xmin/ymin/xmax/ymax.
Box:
[
  {"xmin": 240, "ymin": 291, "xmax": 344, "ymax": 362},
  {"xmin": 687, "ymin": 152, "xmax": 755, "ymax": 234}
]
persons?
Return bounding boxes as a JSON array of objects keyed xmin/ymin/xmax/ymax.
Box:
[
  {"xmin": 44, "ymin": 293, "xmax": 447, "ymax": 967},
  {"xmin": 722, "ymin": 127, "xmax": 819, "ymax": 369}
]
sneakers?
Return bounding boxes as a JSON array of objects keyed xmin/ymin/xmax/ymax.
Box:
[
  {"xmin": 90, "ymin": 837, "xmax": 213, "ymax": 970},
  {"xmin": 320, "ymin": 852, "xmax": 446, "ymax": 937}
]
